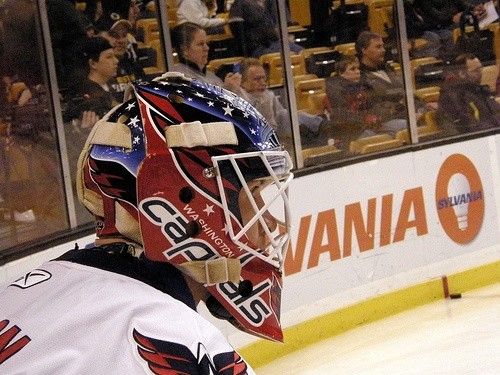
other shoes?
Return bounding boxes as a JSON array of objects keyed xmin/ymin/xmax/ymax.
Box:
[
  {"xmin": 299, "ymin": 123, "xmax": 315, "ymax": 141},
  {"xmin": 319, "ymin": 121, "xmax": 343, "ymax": 138}
]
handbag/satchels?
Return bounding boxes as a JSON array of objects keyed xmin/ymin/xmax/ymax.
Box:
[{"xmin": 455, "ymin": 10, "xmax": 497, "ymax": 62}]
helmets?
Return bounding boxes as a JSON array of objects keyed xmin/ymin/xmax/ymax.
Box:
[{"xmin": 77, "ymin": 71, "xmax": 294, "ymax": 344}]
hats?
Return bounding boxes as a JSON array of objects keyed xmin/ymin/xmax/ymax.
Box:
[
  {"xmin": 96, "ymin": 10, "xmax": 134, "ymax": 33},
  {"xmin": 78, "ymin": 35, "xmax": 113, "ymax": 60}
]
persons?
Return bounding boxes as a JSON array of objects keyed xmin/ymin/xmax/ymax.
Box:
[
  {"xmin": 0, "ymin": 73, "xmax": 296, "ymax": 374},
  {"xmin": 0, "ymin": 0, "xmax": 499, "ymax": 246}
]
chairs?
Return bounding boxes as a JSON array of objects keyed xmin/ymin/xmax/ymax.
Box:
[{"xmin": 0, "ymin": 0, "xmax": 500, "ymax": 229}]
